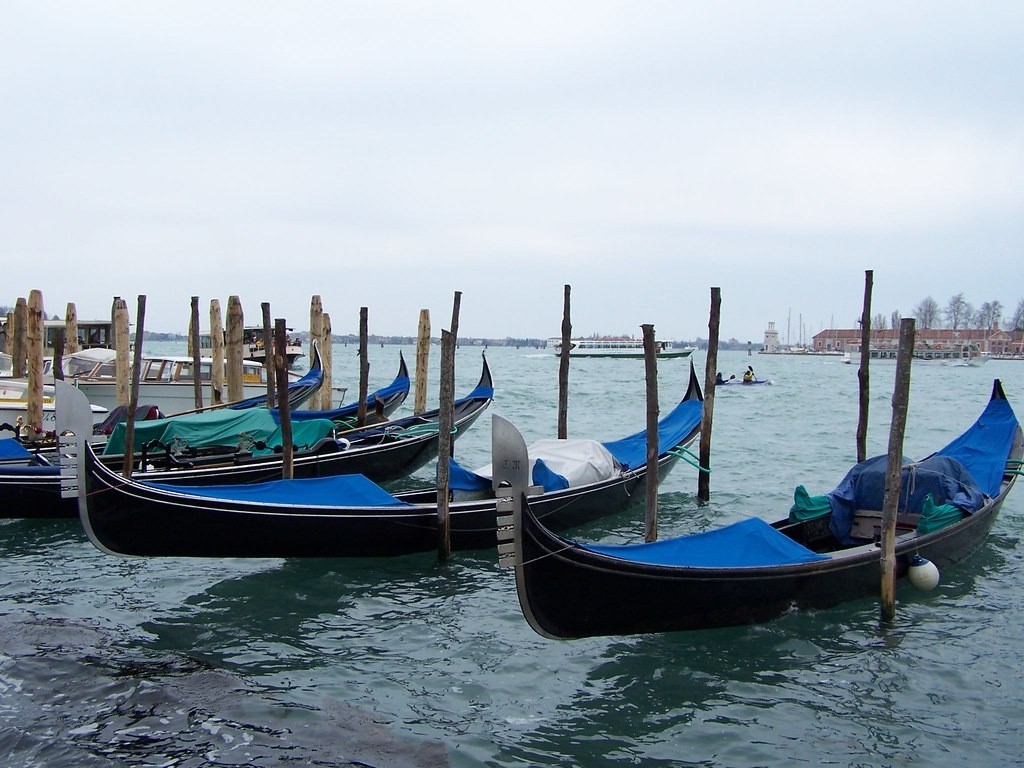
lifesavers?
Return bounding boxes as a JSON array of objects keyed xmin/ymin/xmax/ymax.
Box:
[
  {"xmin": 918, "ymin": 344, "xmax": 922, "ymax": 349},
  {"xmin": 936, "ymin": 346, "xmax": 941, "ymax": 350},
  {"xmin": 880, "ymin": 344, "xmax": 886, "ymax": 349}
]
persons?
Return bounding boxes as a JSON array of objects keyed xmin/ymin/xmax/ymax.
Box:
[
  {"xmin": 716, "ymin": 372, "xmax": 729, "ymax": 384},
  {"xmin": 744, "ymin": 371, "xmax": 754, "ymax": 383},
  {"xmin": 293, "ymin": 338, "xmax": 302, "ymax": 347}
]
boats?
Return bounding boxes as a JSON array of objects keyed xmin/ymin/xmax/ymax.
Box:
[
  {"xmin": 841, "ymin": 340, "xmax": 991, "ymax": 368},
  {"xmin": 553, "ymin": 340, "xmax": 695, "ymax": 358},
  {"xmin": 199, "ymin": 326, "xmax": 306, "ymax": 369},
  {"xmin": 727, "ymin": 379, "xmax": 777, "ymax": 386},
  {"xmin": 0, "ymin": 318, "xmax": 494, "ymax": 519},
  {"xmin": 51, "ymin": 360, "xmax": 709, "ymax": 562},
  {"xmin": 491, "ymin": 379, "xmax": 1023, "ymax": 642}
]
